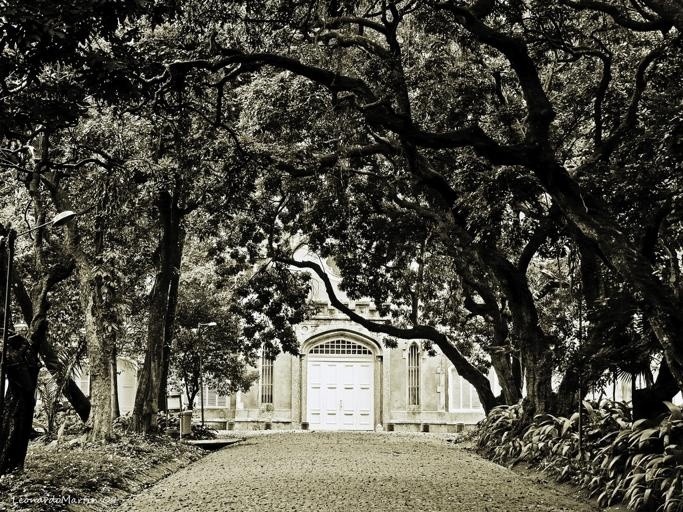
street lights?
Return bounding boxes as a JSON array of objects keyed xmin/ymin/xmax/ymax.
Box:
[
  {"xmin": 0, "ymin": 210, "xmax": 78, "ymax": 416},
  {"xmin": 196, "ymin": 321, "xmax": 217, "ymax": 428}
]
